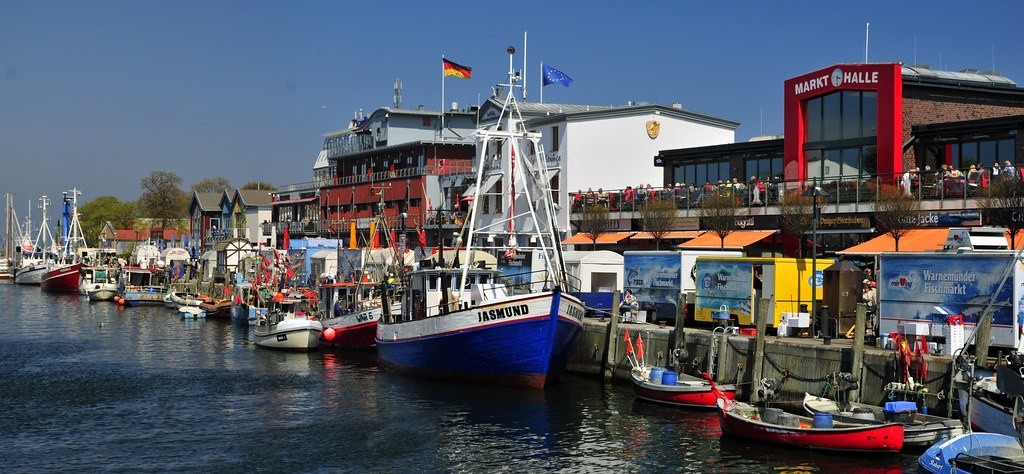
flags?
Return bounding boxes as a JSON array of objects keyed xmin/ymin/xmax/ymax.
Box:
[
  {"xmin": 442, "ymin": 57, "xmax": 472, "ymax": 80},
  {"xmin": 542, "ymin": 64, "xmax": 574, "ymax": 88}
]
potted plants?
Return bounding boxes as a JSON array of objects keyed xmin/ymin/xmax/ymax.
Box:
[{"xmin": 435, "ymin": 203, "xmax": 443, "ymax": 222}]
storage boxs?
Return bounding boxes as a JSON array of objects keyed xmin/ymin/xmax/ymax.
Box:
[
  {"xmin": 711, "ymin": 311, "xmax": 730, "ymax": 320},
  {"xmin": 944, "ymin": 324, "xmax": 964, "ymax": 356},
  {"xmin": 788, "ymin": 313, "xmax": 810, "ymax": 328},
  {"xmin": 739, "ymin": 328, "xmax": 756, "ymax": 336},
  {"xmin": 633, "ymin": 311, "xmax": 646, "ymax": 323},
  {"xmin": 904, "ymin": 323, "xmax": 930, "ymax": 336}
]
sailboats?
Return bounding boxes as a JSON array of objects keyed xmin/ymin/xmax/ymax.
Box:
[{"xmin": 951, "ymin": 241, "xmax": 1024, "ymax": 439}]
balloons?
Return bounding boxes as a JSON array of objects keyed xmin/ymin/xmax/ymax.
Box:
[
  {"xmin": 273, "ymin": 292, "xmax": 284, "ymax": 303},
  {"xmin": 114, "ymin": 296, "xmax": 125, "ymax": 305},
  {"xmin": 323, "ymin": 327, "xmax": 336, "ymax": 341}
]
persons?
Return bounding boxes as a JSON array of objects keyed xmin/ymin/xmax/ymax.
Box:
[
  {"xmin": 573, "ymin": 176, "xmax": 768, "ymax": 213},
  {"xmin": 900, "ymin": 160, "xmax": 1024, "ymax": 198},
  {"xmin": 334, "ymin": 297, "xmax": 343, "ymax": 317},
  {"xmin": 619, "ymin": 289, "xmax": 639, "ymax": 323},
  {"xmin": 301, "ymin": 216, "xmax": 310, "ymax": 225}
]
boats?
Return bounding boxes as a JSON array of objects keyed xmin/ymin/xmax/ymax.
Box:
[
  {"xmin": 0, "ymin": 187, "xmax": 167, "ymax": 309},
  {"xmin": 716, "ymin": 397, "xmax": 904, "ymax": 455},
  {"xmin": 319, "ymin": 183, "xmax": 401, "ymax": 350},
  {"xmin": 230, "ymin": 282, "xmax": 268, "ymax": 326},
  {"xmin": 373, "ymin": 46, "xmax": 587, "ymax": 391},
  {"xmin": 802, "ymin": 392, "xmax": 964, "ymax": 449},
  {"xmin": 164, "ymin": 290, "xmax": 231, "ymax": 320},
  {"xmin": 917, "ymin": 428, "xmax": 1024, "ymax": 474},
  {"xmin": 253, "ymin": 250, "xmax": 324, "ymax": 350},
  {"xmin": 629, "ymin": 363, "xmax": 737, "ymax": 409}
]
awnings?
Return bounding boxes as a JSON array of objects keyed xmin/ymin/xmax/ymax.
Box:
[
  {"xmin": 629, "ymin": 230, "xmax": 708, "ymax": 239},
  {"xmin": 558, "ymin": 231, "xmax": 638, "ymax": 245},
  {"xmin": 677, "ymin": 229, "xmax": 781, "ymax": 250},
  {"xmin": 835, "ymin": 226, "xmax": 1024, "ymax": 258}
]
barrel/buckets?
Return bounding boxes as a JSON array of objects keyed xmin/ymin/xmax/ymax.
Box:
[
  {"xmin": 813, "ymin": 412, "xmax": 833, "ymax": 428},
  {"xmin": 661, "ymin": 371, "xmax": 676, "ymax": 386},
  {"xmin": 649, "ymin": 367, "xmax": 667, "ymax": 385}
]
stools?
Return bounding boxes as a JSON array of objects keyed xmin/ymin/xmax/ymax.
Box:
[
  {"xmin": 763, "ymin": 408, "xmax": 783, "ymax": 424},
  {"xmin": 777, "ymin": 414, "xmax": 800, "ymax": 427}
]
